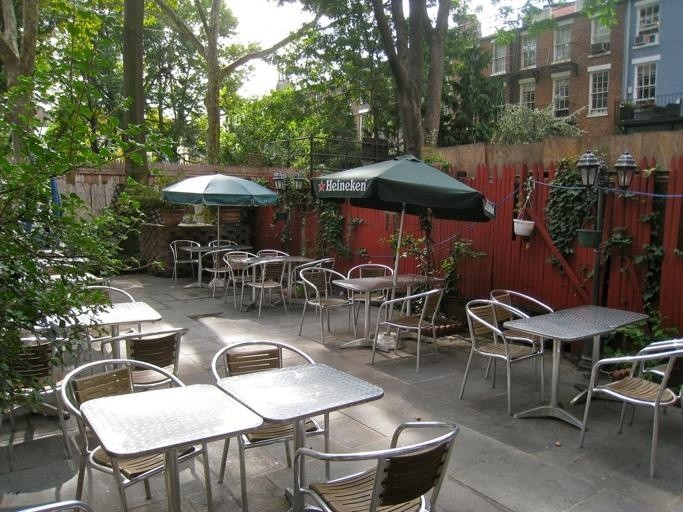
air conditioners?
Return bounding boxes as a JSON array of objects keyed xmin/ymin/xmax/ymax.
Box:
[
  {"xmin": 590, "ymin": 42, "xmax": 607, "ymax": 54},
  {"xmin": 633, "ymin": 35, "xmax": 647, "ymax": 45}
]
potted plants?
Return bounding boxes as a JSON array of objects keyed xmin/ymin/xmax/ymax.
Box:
[
  {"xmin": 618, "ymin": 99, "xmax": 679, "ymax": 121},
  {"xmin": 512, "ymin": 176, "xmax": 535, "ymax": 237},
  {"xmin": 576, "ymin": 201, "xmax": 603, "ymax": 248}
]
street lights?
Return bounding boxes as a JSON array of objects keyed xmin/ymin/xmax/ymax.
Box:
[
  {"xmin": 272, "ymin": 171, "xmax": 306, "ymax": 286},
  {"xmin": 575, "ymin": 147, "xmax": 637, "ymax": 384}
]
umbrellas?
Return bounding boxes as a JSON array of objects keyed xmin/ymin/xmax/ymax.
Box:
[
  {"xmin": 310, "ymin": 154, "xmax": 495, "ymax": 335},
  {"xmin": 162, "ymin": 173, "xmax": 279, "ymax": 280}
]
